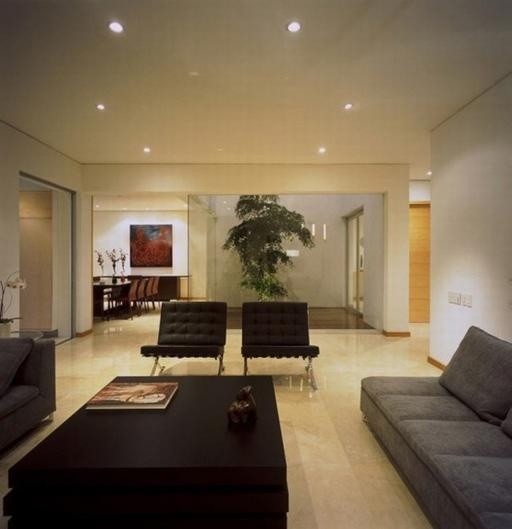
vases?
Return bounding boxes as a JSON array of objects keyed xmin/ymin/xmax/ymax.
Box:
[{"xmin": 0, "ymin": 324, "xmax": 12, "ymax": 340}]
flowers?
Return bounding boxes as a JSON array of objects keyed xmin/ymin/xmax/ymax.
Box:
[{"xmin": 0, "ymin": 270, "xmax": 28, "ymax": 326}]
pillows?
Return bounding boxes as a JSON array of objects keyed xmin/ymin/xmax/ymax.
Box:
[
  {"xmin": 0, "ymin": 338, "xmax": 34, "ymax": 398},
  {"xmin": 437, "ymin": 325, "xmax": 511, "ymax": 426}
]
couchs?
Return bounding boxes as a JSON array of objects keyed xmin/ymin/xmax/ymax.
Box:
[{"xmin": 359, "ymin": 375, "xmax": 512, "ymax": 529}]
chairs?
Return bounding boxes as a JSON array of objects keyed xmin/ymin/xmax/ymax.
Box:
[
  {"xmin": 92, "ymin": 275, "xmax": 160, "ymax": 323},
  {"xmin": 139, "ymin": 301, "xmax": 228, "ymax": 377},
  {"xmin": 242, "ymin": 302, "xmax": 320, "ymax": 391},
  {"xmin": 0, "ymin": 337, "xmax": 55, "ymax": 449}
]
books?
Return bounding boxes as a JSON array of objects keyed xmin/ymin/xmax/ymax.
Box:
[{"xmin": 85, "ymin": 380, "xmax": 180, "ymax": 411}]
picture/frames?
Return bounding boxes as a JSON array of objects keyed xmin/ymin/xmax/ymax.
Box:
[{"xmin": 129, "ymin": 223, "xmax": 173, "ymax": 268}]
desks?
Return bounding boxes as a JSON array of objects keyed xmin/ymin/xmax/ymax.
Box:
[{"xmin": 93, "ymin": 278, "xmax": 131, "ymax": 317}]
